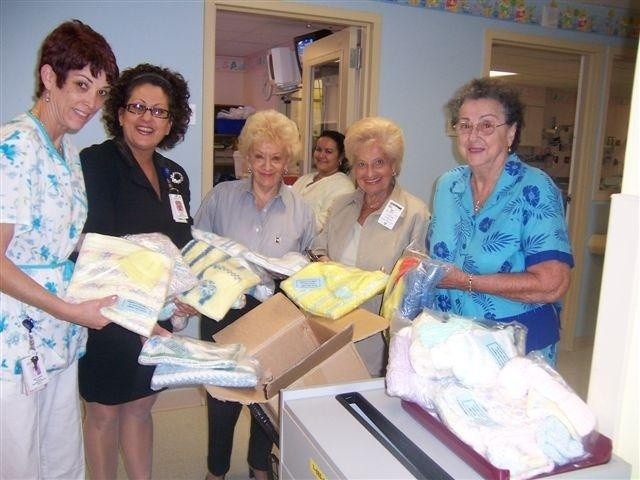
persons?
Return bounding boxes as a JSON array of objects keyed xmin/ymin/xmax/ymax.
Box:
[
  {"xmin": 315, "ymin": 110, "xmax": 431, "ymax": 377},
  {"xmin": 426, "ymin": 76, "xmax": 578, "ymax": 365},
  {"xmin": 78, "ymin": 63, "xmax": 194, "ymax": 480},
  {"xmin": 0, "ymin": 16, "xmax": 120, "ymax": 480},
  {"xmin": 292, "ymin": 129, "xmax": 354, "ymax": 232},
  {"xmin": 193, "ymin": 107, "xmax": 317, "ymax": 480}
]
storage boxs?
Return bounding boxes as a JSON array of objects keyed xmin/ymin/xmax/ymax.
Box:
[
  {"xmin": 217, "ymin": 118, "xmax": 246, "ymax": 135},
  {"xmin": 203, "ymin": 291, "xmax": 390, "ymax": 449}
]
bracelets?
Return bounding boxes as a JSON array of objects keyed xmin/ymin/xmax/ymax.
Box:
[{"xmin": 468, "ymin": 272, "xmax": 474, "ymax": 293}]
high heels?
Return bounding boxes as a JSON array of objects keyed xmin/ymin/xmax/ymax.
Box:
[{"xmin": 249, "ymin": 468, "xmax": 270, "ymax": 480}]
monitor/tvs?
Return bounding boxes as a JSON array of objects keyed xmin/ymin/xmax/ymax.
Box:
[
  {"xmin": 293, "ymin": 27, "xmax": 333, "ymax": 78},
  {"xmin": 263, "ymin": 46, "xmax": 302, "ymax": 96}
]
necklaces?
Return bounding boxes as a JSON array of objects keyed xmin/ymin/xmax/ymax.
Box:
[{"xmin": 471, "ymin": 200, "xmax": 483, "ymax": 213}]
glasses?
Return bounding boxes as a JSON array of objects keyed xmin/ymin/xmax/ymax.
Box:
[
  {"xmin": 452, "ymin": 122, "xmax": 507, "ymax": 136},
  {"xmin": 121, "ymin": 103, "xmax": 169, "ymax": 120}
]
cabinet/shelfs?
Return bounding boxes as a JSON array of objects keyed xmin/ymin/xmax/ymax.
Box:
[
  {"xmin": 278, "ymin": 376, "xmax": 631, "ymax": 480},
  {"xmin": 518, "ymin": 106, "xmax": 545, "ymax": 147}
]
